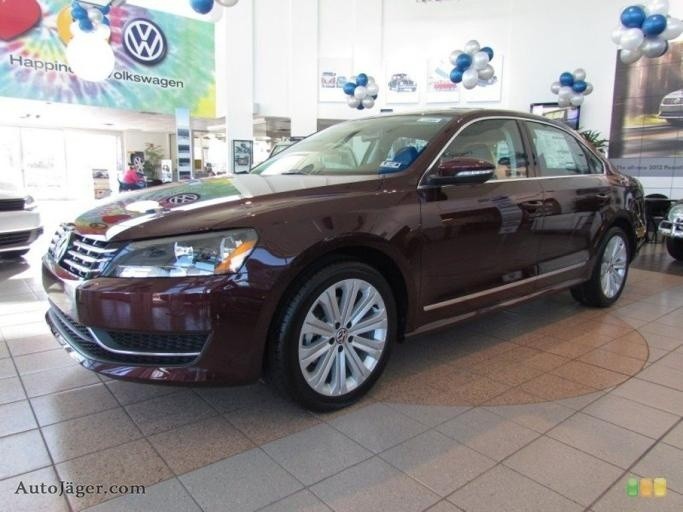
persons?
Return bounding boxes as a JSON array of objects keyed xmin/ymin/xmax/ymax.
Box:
[{"xmin": 121, "ymin": 165, "xmax": 145, "ymax": 192}]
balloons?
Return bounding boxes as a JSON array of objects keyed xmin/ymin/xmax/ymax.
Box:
[
  {"xmin": 339, "ymin": 72, "xmax": 381, "ymax": 113},
  {"xmin": 448, "ymin": 38, "xmax": 494, "ymax": 91},
  {"xmin": 608, "ymin": 0, "xmax": 683, "ymax": 66},
  {"xmin": 70, "ymin": 1, "xmax": 112, "ymax": 43},
  {"xmin": 215, "ymin": 1, "xmax": 241, "ymax": 8},
  {"xmin": 548, "ymin": 66, "xmax": 594, "ymax": 110},
  {"xmin": 189, "ymin": 0, "xmax": 216, "ymax": 15},
  {"xmin": 378, "ymin": 159, "xmax": 405, "ymax": 174}
]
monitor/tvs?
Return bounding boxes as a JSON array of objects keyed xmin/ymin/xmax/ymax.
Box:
[{"xmin": 530, "ymin": 103, "xmax": 581, "ymax": 131}]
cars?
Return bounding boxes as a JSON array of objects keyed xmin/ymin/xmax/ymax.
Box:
[
  {"xmin": 659, "ymin": 205, "xmax": 682, "ymax": 261},
  {"xmin": 0, "ymin": 181, "xmax": 42, "ymax": 263},
  {"xmin": 654, "ymin": 89, "xmax": 683, "ymax": 126},
  {"xmin": 40, "ymin": 107, "xmax": 649, "ymax": 412}
]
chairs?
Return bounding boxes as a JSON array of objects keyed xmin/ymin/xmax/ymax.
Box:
[{"xmin": 461, "ymin": 144, "xmax": 497, "ymax": 178}]
